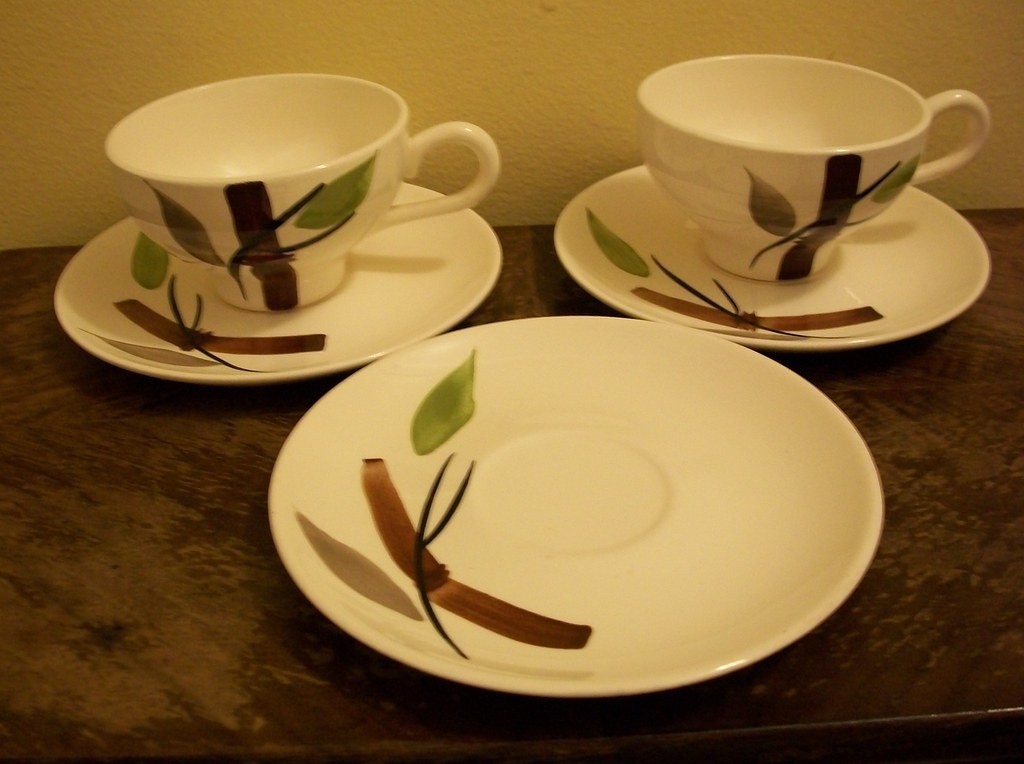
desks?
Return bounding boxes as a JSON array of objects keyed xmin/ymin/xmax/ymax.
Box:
[{"xmin": 2, "ymin": 206, "xmax": 1024, "ymax": 764}]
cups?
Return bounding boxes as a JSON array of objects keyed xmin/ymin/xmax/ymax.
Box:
[
  {"xmin": 105, "ymin": 73, "xmax": 500, "ymax": 312},
  {"xmin": 637, "ymin": 54, "xmax": 992, "ymax": 281}
]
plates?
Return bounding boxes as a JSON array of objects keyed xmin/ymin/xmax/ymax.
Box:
[
  {"xmin": 554, "ymin": 163, "xmax": 991, "ymax": 350},
  {"xmin": 267, "ymin": 316, "xmax": 885, "ymax": 698},
  {"xmin": 53, "ymin": 180, "xmax": 503, "ymax": 383}
]
